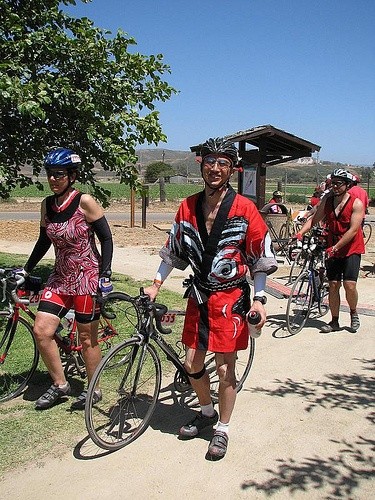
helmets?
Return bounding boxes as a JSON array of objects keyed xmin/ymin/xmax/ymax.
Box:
[
  {"xmin": 44, "ymin": 148, "xmax": 82, "ymax": 169},
  {"xmin": 273, "ymin": 191, "xmax": 281, "ymax": 195},
  {"xmin": 200, "ymin": 137, "xmax": 238, "ymax": 168},
  {"xmin": 325, "ymin": 168, "xmax": 360, "ymax": 183}
]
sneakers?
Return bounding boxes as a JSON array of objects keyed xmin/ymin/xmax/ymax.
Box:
[
  {"xmin": 350, "ymin": 312, "xmax": 360, "ymax": 331},
  {"xmin": 320, "ymin": 321, "xmax": 339, "ymax": 331},
  {"xmin": 209, "ymin": 430, "xmax": 228, "ymax": 458},
  {"xmin": 34, "ymin": 381, "xmax": 71, "ymax": 408},
  {"xmin": 179, "ymin": 409, "xmax": 218, "ymax": 437},
  {"xmin": 71, "ymin": 388, "xmax": 102, "ymax": 409}
]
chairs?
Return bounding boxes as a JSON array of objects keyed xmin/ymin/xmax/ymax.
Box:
[{"xmin": 267, "ymin": 222, "xmax": 291, "ymax": 265}]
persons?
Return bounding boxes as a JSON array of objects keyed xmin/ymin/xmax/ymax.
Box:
[
  {"xmin": 0, "ymin": 147, "xmax": 113, "ymax": 409},
  {"xmin": 141, "ymin": 138, "xmax": 278, "ymax": 456},
  {"xmin": 305, "ymin": 174, "xmax": 369, "ymax": 246},
  {"xmin": 288, "ymin": 168, "xmax": 365, "ymax": 332},
  {"xmin": 268, "ymin": 191, "xmax": 283, "ymax": 214}
]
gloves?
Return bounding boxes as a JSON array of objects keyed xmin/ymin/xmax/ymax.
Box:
[
  {"xmin": 97, "ymin": 277, "xmax": 113, "ymax": 297},
  {"xmin": 291, "ymin": 233, "xmax": 303, "ymax": 240},
  {"xmin": 6, "ymin": 268, "xmax": 28, "ymax": 286},
  {"xmin": 325, "ymin": 246, "xmax": 339, "ymax": 260}
]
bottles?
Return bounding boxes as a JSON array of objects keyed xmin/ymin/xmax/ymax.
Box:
[
  {"xmin": 315, "ymin": 270, "xmax": 321, "ymax": 287},
  {"xmin": 247, "ymin": 310, "xmax": 261, "ymax": 339}
]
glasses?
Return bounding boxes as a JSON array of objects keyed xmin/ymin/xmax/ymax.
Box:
[
  {"xmin": 330, "ymin": 181, "xmax": 347, "ymax": 187},
  {"xmin": 46, "ymin": 167, "xmax": 69, "ymax": 178},
  {"xmin": 275, "ymin": 196, "xmax": 281, "ymax": 198},
  {"xmin": 201, "ymin": 155, "xmax": 232, "ymax": 168}
]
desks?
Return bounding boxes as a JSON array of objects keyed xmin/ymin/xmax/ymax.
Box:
[{"xmin": 153, "ymin": 224, "xmax": 172, "ymax": 231}]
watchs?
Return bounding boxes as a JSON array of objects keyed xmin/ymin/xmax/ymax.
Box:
[{"xmin": 253, "ymin": 295, "xmax": 267, "ymax": 304}]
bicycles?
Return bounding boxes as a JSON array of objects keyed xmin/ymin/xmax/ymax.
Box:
[
  {"xmin": 84, "ymin": 286, "xmax": 255, "ymax": 452},
  {"xmin": 362, "ymin": 224, "xmax": 372, "ymax": 245},
  {"xmin": 289, "ymin": 235, "xmax": 330, "ymax": 285},
  {"xmin": 0, "ymin": 266, "xmax": 142, "ymax": 403},
  {"xmin": 286, "ymin": 235, "xmax": 331, "ymax": 335}
]
